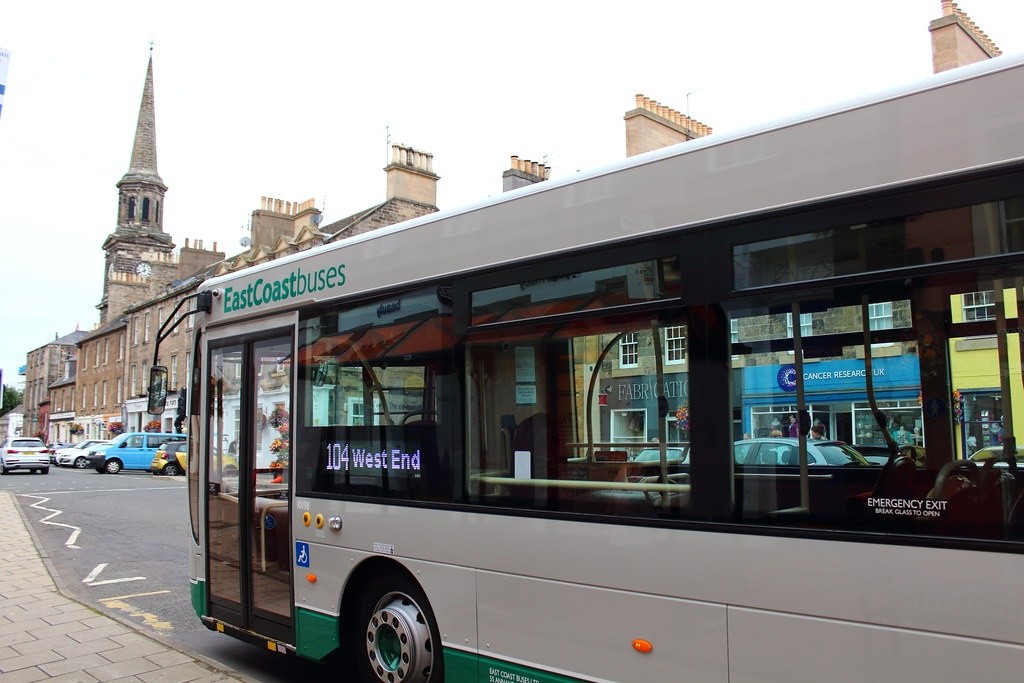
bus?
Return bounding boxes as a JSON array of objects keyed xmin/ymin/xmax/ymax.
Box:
[{"xmin": 150, "ymin": 49, "xmax": 1024, "ymax": 683}]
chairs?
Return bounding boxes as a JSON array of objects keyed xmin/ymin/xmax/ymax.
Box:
[{"xmin": 506, "ymin": 415, "xmax": 1024, "ymax": 547}]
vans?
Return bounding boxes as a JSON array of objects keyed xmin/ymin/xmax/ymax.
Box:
[{"xmin": 88, "ymin": 433, "xmax": 188, "ymax": 473}]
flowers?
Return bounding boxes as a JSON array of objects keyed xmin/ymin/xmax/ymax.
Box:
[
  {"xmin": 69, "ymin": 424, "xmax": 83, "ymax": 435},
  {"xmin": 255, "ymin": 413, "xmax": 267, "ymax": 431},
  {"xmin": 953, "ymin": 388, "xmax": 965, "ymax": 425},
  {"xmin": 108, "ymin": 421, "xmax": 124, "ymax": 435},
  {"xmin": 673, "ymin": 401, "xmax": 690, "ymax": 432},
  {"xmin": 268, "ymin": 406, "xmax": 292, "ymax": 468},
  {"xmin": 144, "ymin": 420, "xmax": 162, "ymax": 432},
  {"xmin": 917, "ymin": 391, "xmax": 924, "ymax": 408}
]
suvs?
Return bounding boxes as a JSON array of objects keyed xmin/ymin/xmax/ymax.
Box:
[
  {"xmin": 631, "ymin": 435, "xmax": 1021, "ymax": 472},
  {"xmin": 0, "ymin": 437, "xmax": 51, "ymax": 475}
]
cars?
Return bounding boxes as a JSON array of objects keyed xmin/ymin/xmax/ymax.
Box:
[
  {"xmin": 49, "ymin": 439, "xmax": 113, "ymax": 468},
  {"xmin": 151, "ymin": 442, "xmax": 239, "ymax": 476}
]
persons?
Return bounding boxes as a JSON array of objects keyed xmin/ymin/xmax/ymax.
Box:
[
  {"xmin": 811, "ymin": 424, "xmax": 828, "ymax": 442},
  {"xmin": 770, "ymin": 417, "xmax": 781, "ymax": 432},
  {"xmin": 788, "ymin": 417, "xmax": 798, "ymax": 437}
]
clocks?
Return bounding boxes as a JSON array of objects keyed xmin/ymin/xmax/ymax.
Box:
[{"xmin": 134, "ymin": 260, "xmax": 152, "ymax": 280}]
